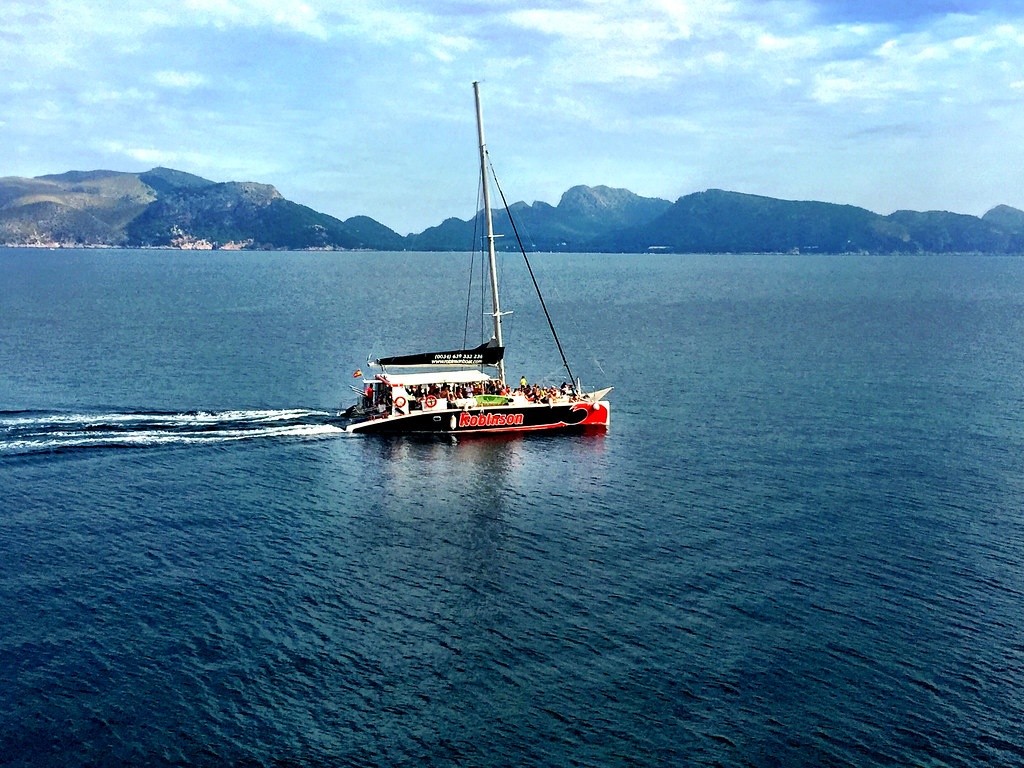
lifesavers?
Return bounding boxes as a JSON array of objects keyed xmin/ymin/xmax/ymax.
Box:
[
  {"xmin": 395, "ymin": 396, "xmax": 407, "ymax": 408},
  {"xmin": 425, "ymin": 395, "xmax": 437, "ymax": 408}
]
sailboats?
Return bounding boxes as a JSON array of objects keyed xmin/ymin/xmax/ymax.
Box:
[{"xmin": 337, "ymin": 74, "xmax": 614, "ymax": 434}]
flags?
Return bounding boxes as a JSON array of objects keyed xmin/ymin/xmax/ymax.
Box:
[{"xmin": 353, "ymin": 369, "xmax": 362, "ymax": 378}]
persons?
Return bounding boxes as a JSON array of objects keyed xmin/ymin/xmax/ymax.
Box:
[{"xmin": 365, "ymin": 375, "xmax": 569, "ymax": 414}]
